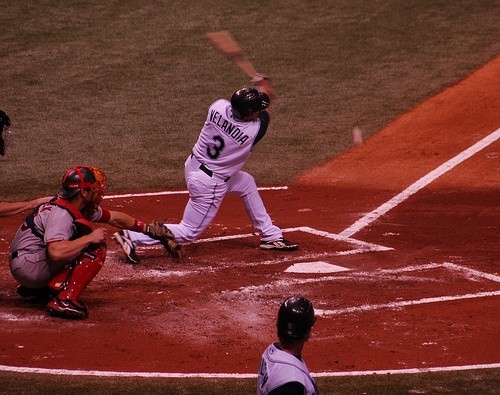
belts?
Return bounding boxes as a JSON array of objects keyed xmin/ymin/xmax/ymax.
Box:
[
  {"xmin": 11, "ymin": 251, "xmax": 18, "ymax": 260},
  {"xmin": 190, "ymin": 153, "xmax": 229, "ymax": 182}
]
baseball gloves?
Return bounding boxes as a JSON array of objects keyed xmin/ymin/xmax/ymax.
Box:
[{"xmin": 149, "ymin": 221, "xmax": 184, "ymax": 262}]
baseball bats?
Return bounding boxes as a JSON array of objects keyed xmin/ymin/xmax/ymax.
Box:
[{"xmin": 204, "ymin": 30, "xmax": 257, "ymax": 78}]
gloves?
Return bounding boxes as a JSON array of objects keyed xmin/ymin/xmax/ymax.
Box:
[{"xmin": 250, "ymin": 72, "xmax": 269, "ymax": 83}]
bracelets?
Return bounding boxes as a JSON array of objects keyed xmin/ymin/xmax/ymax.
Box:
[{"xmin": 131, "ymin": 219, "xmax": 144, "ymax": 233}]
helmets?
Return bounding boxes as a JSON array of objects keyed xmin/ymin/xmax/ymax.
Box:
[
  {"xmin": 231, "ymin": 87, "xmax": 270, "ymax": 118},
  {"xmin": 277, "ymin": 296, "xmax": 315, "ymax": 335},
  {"xmin": 56, "ymin": 165, "xmax": 96, "ymax": 198}
]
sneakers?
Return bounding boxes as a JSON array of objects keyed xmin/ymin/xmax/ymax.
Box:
[
  {"xmin": 45, "ymin": 297, "xmax": 87, "ymax": 318},
  {"xmin": 16, "ymin": 284, "xmax": 58, "ymax": 299},
  {"xmin": 260, "ymin": 236, "xmax": 299, "ymax": 250},
  {"xmin": 114, "ymin": 230, "xmax": 140, "ymax": 263}
]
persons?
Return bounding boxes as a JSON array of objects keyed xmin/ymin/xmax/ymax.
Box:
[
  {"xmin": 10, "ymin": 166, "xmax": 183, "ymax": 316},
  {"xmin": 0, "ymin": 109, "xmax": 54, "ymax": 216},
  {"xmin": 256, "ymin": 296, "xmax": 319, "ymax": 395},
  {"xmin": 114, "ymin": 72, "xmax": 299, "ymax": 263}
]
